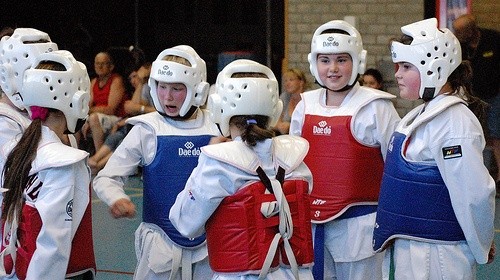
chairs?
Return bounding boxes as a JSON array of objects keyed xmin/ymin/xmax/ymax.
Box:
[{"xmin": 110, "ymin": 46, "xmax": 146, "ymax": 99}]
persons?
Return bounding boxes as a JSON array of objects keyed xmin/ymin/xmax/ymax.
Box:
[
  {"xmin": 168, "ymin": 58, "xmax": 315, "ymax": 280},
  {"xmin": 62, "ymin": 51, "xmax": 157, "ymax": 171},
  {"xmin": 273, "ymin": 68, "xmax": 313, "ymax": 137},
  {"xmin": 92, "ymin": 46, "xmax": 223, "ymax": 280},
  {"xmin": 451, "ymin": 13, "xmax": 500, "ymax": 138},
  {"xmin": 288, "ymin": 20, "xmax": 402, "ymax": 280},
  {"xmin": 371, "ymin": 17, "xmax": 496, "ymax": 280},
  {"xmin": 363, "ymin": 69, "xmax": 383, "ymax": 91},
  {"xmin": 1, "ymin": 27, "xmax": 97, "ymax": 280}
]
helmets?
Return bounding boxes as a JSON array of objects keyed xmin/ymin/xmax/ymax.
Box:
[
  {"xmin": 0, "ymin": 28, "xmax": 59, "ymax": 110},
  {"xmin": 208, "ymin": 59, "xmax": 283, "ymax": 137},
  {"xmin": 148, "ymin": 44, "xmax": 209, "ymax": 117},
  {"xmin": 391, "ymin": 17, "xmax": 462, "ymax": 101},
  {"xmin": 23, "ymin": 50, "xmax": 90, "ymax": 134},
  {"xmin": 308, "ymin": 20, "xmax": 367, "ymax": 86}
]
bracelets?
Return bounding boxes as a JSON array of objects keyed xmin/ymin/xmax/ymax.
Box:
[{"xmin": 140, "ymin": 105, "xmax": 145, "ymax": 113}]
